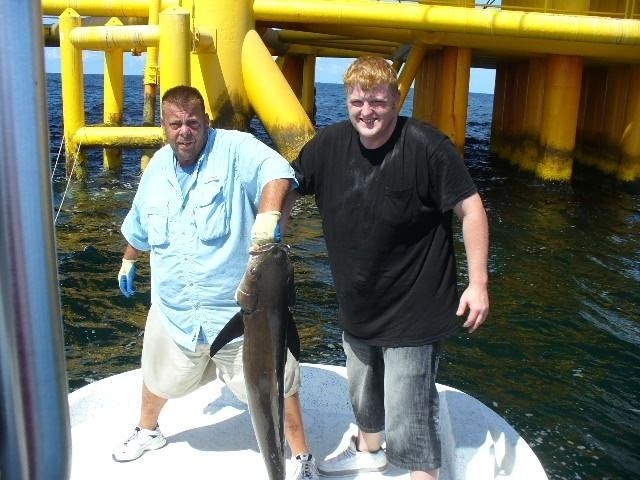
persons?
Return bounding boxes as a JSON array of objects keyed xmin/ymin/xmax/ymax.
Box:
[
  {"xmin": 111, "ymin": 87, "xmax": 319, "ymax": 478},
  {"xmin": 273, "ymin": 55, "xmax": 490, "ymax": 479}
]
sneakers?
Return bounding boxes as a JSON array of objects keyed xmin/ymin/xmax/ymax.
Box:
[
  {"xmin": 294, "ymin": 453, "xmax": 318, "ymax": 479},
  {"xmin": 113, "ymin": 424, "xmax": 167, "ymax": 462},
  {"xmin": 317, "ymin": 437, "xmax": 388, "ymax": 477}
]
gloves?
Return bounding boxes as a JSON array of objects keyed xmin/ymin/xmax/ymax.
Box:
[
  {"xmin": 251, "ymin": 211, "xmax": 281, "ymax": 245},
  {"xmin": 119, "ymin": 258, "xmax": 139, "ymax": 297}
]
